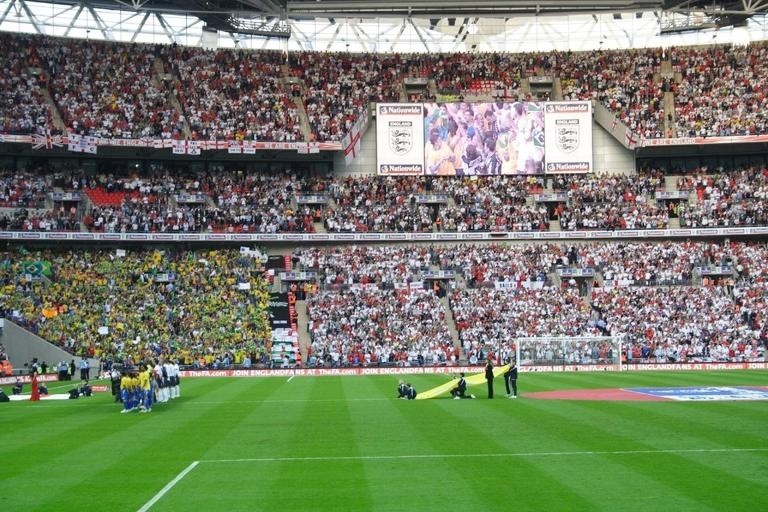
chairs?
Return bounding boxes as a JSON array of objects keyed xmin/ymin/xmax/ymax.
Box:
[{"xmin": 0, "ymin": 25, "xmax": 768, "ymax": 372}]
